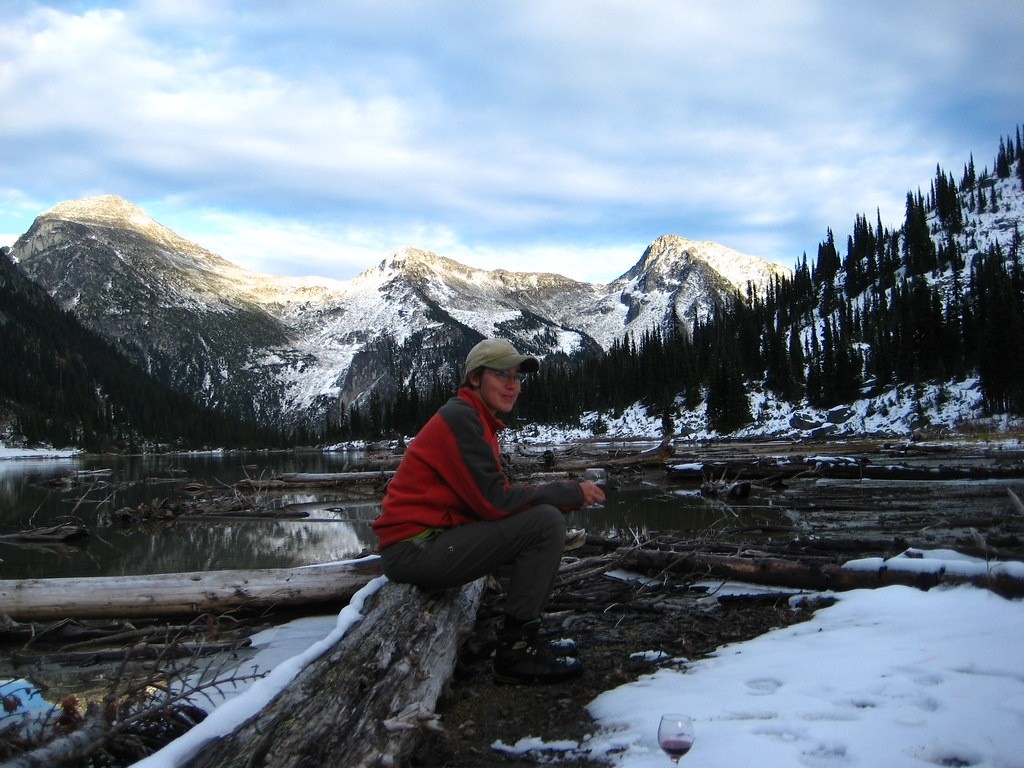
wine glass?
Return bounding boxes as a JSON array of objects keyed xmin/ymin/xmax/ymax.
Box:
[
  {"xmin": 658, "ymin": 714, "xmax": 694, "ymax": 768},
  {"xmin": 584, "ymin": 469, "xmax": 606, "ymax": 508}
]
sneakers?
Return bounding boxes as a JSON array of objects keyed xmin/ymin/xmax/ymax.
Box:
[
  {"xmin": 542, "ymin": 638, "xmax": 577, "ymax": 657},
  {"xmin": 493, "ymin": 618, "xmax": 583, "ymax": 684}
]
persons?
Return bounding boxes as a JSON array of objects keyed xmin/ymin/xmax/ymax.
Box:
[{"xmin": 370, "ymin": 336, "xmax": 606, "ymax": 687}]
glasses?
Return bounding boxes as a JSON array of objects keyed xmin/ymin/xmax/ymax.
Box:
[{"xmin": 484, "ymin": 367, "xmax": 527, "ymax": 384}]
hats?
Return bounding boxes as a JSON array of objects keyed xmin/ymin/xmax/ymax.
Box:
[{"xmin": 465, "ymin": 338, "xmax": 539, "ymax": 376}]
